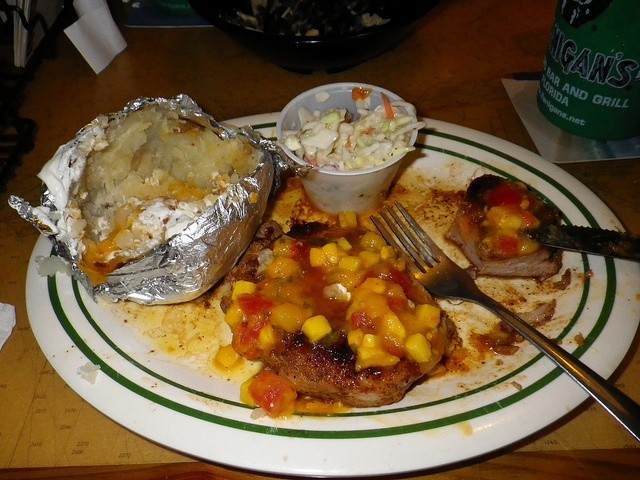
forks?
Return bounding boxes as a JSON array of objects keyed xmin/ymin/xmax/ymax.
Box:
[{"xmin": 368, "ymin": 200, "xmax": 640, "ymax": 441}]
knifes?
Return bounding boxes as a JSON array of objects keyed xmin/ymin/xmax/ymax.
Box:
[{"xmin": 530, "ymin": 224, "xmax": 640, "ymax": 265}]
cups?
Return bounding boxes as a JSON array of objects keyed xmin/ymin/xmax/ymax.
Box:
[{"xmin": 275, "ymin": 81, "xmax": 419, "ymax": 215}]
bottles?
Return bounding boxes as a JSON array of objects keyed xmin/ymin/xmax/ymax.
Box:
[{"xmin": 536, "ymin": 0, "xmax": 639, "ymax": 141}]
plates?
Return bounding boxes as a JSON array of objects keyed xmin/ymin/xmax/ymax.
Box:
[{"xmin": 25, "ymin": 111, "xmax": 639, "ymax": 479}]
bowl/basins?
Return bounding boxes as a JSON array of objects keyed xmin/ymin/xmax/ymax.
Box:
[{"xmin": 187, "ymin": 0, "xmax": 446, "ymax": 76}]
